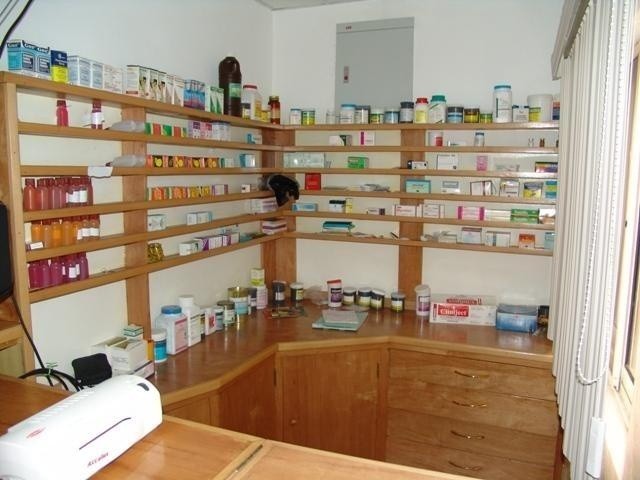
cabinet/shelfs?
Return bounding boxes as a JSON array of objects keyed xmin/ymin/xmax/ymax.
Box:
[{"xmin": 1, "ymin": 73, "xmax": 561, "ymax": 480}]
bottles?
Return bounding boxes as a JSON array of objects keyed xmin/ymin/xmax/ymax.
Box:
[
  {"xmin": 90, "ymin": 99, "xmax": 104, "ymax": 129},
  {"xmin": 20, "ymin": 177, "xmax": 100, "ymax": 292},
  {"xmin": 539, "ymin": 137, "xmax": 545, "ymax": 146},
  {"xmin": 219, "ymin": 55, "xmax": 555, "ymax": 122},
  {"xmin": 473, "ymin": 131, "xmax": 485, "ymax": 147},
  {"xmin": 326, "ymin": 278, "xmax": 431, "ymax": 317},
  {"xmin": 150, "ymin": 281, "xmax": 304, "ymax": 364},
  {"xmin": 55, "ymin": 99, "xmax": 69, "ymax": 126},
  {"xmin": 528, "ymin": 137, "xmax": 534, "ymax": 147}
]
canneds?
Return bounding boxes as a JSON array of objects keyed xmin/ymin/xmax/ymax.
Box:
[
  {"xmin": 301, "ymin": 109, "xmax": 316, "ymax": 126},
  {"xmin": 289, "ymin": 283, "xmax": 304, "ymax": 301},
  {"xmin": 391, "ymin": 292, "xmax": 405, "ymax": 312},
  {"xmin": 343, "ymin": 287, "xmax": 385, "ymax": 310},
  {"xmin": 214, "ymin": 286, "xmax": 256, "ymax": 331},
  {"xmin": 447, "ymin": 106, "xmax": 492, "ymax": 123},
  {"xmin": 355, "ymin": 101, "xmax": 414, "ymax": 124}
]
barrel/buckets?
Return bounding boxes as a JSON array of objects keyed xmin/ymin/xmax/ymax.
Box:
[
  {"xmin": 526, "ymin": 94, "xmax": 553, "ymax": 122},
  {"xmin": 218, "ymin": 56, "xmax": 242, "ymax": 116}
]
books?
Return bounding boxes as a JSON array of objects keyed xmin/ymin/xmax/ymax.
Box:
[{"xmin": 312, "ymin": 309, "xmax": 368, "ymax": 331}]
